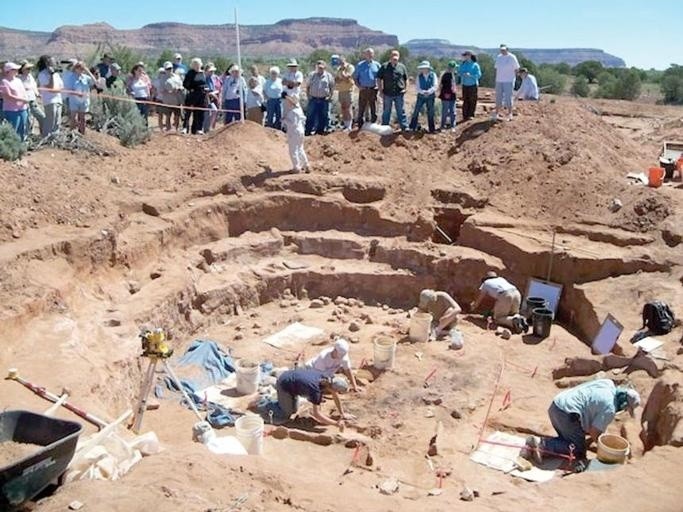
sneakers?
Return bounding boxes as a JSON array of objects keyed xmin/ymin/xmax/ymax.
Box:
[
  {"xmin": 513, "ymin": 317, "xmax": 528, "ymax": 334},
  {"xmin": 527, "ymin": 436, "xmax": 542, "ymax": 464}
]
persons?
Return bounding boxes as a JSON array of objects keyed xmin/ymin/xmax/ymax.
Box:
[
  {"xmin": 468, "ymin": 271, "xmax": 528, "ymax": 335},
  {"xmin": 352, "ymin": 48, "xmax": 382, "ymax": 129},
  {"xmin": 436, "ymin": 60, "xmax": 458, "ymax": 130},
  {"xmin": 277, "ymin": 93, "xmax": 310, "ymax": 173},
  {"xmin": 418, "ymin": 288, "xmax": 465, "ymax": 349},
  {"xmin": 152, "ymin": 54, "xmax": 247, "ymax": 135},
  {"xmin": 377, "ymin": 49, "xmax": 407, "ymax": 131},
  {"xmin": 512, "ymin": 67, "xmax": 539, "ymax": 101},
  {"xmin": 89, "ymin": 52, "xmax": 152, "ymax": 128},
  {"xmin": 247, "ymin": 368, "xmax": 358, "ymax": 426},
  {"xmin": 490, "ymin": 43, "xmax": 519, "ymax": 123},
  {"xmin": 304, "ymin": 338, "xmax": 363, "ymax": 393},
  {"xmin": 331, "ymin": 55, "xmax": 354, "ymax": 131},
  {"xmin": 405, "ymin": 60, "xmax": 438, "ymax": 133},
  {"xmin": 455, "ymin": 50, "xmax": 482, "ymax": 121},
  {"xmin": 519, "ymin": 378, "xmax": 642, "ymax": 473},
  {"xmin": 245, "ymin": 58, "xmax": 335, "ymax": 137},
  {"xmin": 1, "ymin": 55, "xmax": 96, "ymax": 142}
]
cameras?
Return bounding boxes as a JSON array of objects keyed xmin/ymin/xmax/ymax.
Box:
[{"xmin": 51, "ymin": 66, "xmax": 63, "ymax": 73}]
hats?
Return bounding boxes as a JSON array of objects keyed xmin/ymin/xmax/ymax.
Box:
[
  {"xmin": 228, "ymin": 66, "xmax": 244, "ymax": 75},
  {"xmin": 335, "ymin": 339, "xmax": 347, "ymax": 359},
  {"xmin": 286, "ymin": 59, "xmax": 298, "ymax": 67},
  {"xmin": 158, "ymin": 54, "xmax": 217, "ymax": 73},
  {"xmin": 331, "ymin": 377, "xmax": 347, "ymax": 392},
  {"xmin": 482, "ymin": 271, "xmax": 497, "ymax": 282},
  {"xmin": 3, "ymin": 59, "xmax": 33, "ymax": 72},
  {"xmin": 416, "ymin": 51, "xmax": 470, "ymax": 69},
  {"xmin": 626, "ymin": 389, "xmax": 640, "ymax": 419},
  {"xmin": 420, "ymin": 289, "xmax": 433, "ymax": 309},
  {"xmin": 104, "ymin": 53, "xmax": 122, "ymax": 71}
]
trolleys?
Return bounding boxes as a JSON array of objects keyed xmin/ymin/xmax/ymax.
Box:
[{"xmin": 1, "ymin": 408, "xmax": 85, "ymax": 512}]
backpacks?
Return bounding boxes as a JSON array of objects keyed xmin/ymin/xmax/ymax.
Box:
[{"xmin": 643, "ymin": 301, "xmax": 674, "ymax": 333}]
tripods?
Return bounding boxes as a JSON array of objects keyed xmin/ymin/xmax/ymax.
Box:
[{"xmin": 131, "ymin": 358, "xmax": 203, "ymax": 435}]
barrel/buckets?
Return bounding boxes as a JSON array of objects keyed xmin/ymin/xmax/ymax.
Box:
[
  {"xmin": 236, "ymin": 359, "xmax": 261, "ymax": 394},
  {"xmin": 649, "ymin": 166, "xmax": 667, "ymax": 187},
  {"xmin": 375, "ymin": 337, "xmax": 395, "ymax": 370},
  {"xmin": 532, "ymin": 307, "xmax": 553, "ymax": 338},
  {"xmin": 235, "ymin": 415, "xmax": 263, "ymax": 456},
  {"xmin": 409, "ymin": 312, "xmax": 433, "ymax": 342},
  {"xmin": 660, "ymin": 158, "xmax": 675, "ymax": 179},
  {"xmin": 597, "ymin": 433, "xmax": 629, "ymax": 465},
  {"xmin": 526, "ymin": 296, "xmax": 545, "ymax": 323}
]
